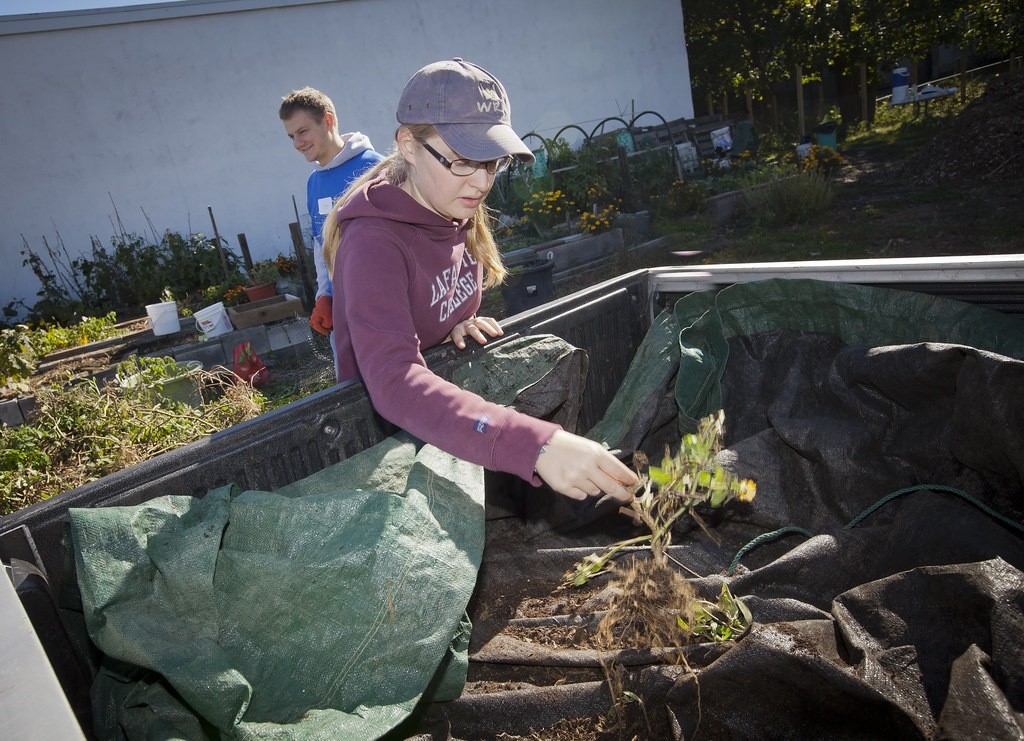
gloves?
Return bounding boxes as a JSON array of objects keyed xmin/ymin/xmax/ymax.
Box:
[{"xmin": 311, "ymin": 295, "xmax": 333, "ymax": 336}]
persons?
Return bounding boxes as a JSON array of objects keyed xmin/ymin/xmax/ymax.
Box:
[
  {"xmin": 280, "ymin": 85, "xmax": 389, "ymax": 387},
  {"xmin": 320, "ymin": 55, "xmax": 639, "ymax": 505}
]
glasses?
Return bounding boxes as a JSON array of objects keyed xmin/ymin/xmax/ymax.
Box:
[{"xmin": 414, "ymin": 135, "xmax": 512, "ymax": 176}]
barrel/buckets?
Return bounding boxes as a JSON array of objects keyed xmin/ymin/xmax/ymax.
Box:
[
  {"xmin": 145, "ymin": 301, "xmax": 180, "ymax": 335},
  {"xmin": 119, "ymin": 361, "xmax": 202, "ymax": 412},
  {"xmin": 193, "ymin": 301, "xmax": 233, "ymax": 337},
  {"xmin": 499, "ymin": 258, "xmax": 556, "ymax": 316}
]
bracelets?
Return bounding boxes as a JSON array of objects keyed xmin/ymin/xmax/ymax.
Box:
[{"xmin": 533, "ymin": 437, "xmax": 551, "ymax": 472}]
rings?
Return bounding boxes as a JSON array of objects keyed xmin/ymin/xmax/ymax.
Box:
[{"xmin": 467, "ymin": 325, "xmax": 478, "ymax": 332}]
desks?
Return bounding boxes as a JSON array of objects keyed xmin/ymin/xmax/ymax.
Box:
[{"xmin": 891, "ymin": 87, "xmax": 957, "ymax": 131}]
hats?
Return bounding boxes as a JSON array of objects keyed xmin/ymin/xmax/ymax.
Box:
[{"xmin": 396, "ymin": 57, "xmax": 536, "ymax": 165}]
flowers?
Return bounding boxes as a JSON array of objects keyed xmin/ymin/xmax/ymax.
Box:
[{"xmin": 250, "ymin": 259, "xmax": 280, "ymax": 285}]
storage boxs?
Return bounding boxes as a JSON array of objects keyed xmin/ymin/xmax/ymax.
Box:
[{"xmin": 227, "ymin": 292, "xmax": 303, "ymax": 328}]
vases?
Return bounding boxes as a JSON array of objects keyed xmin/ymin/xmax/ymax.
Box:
[{"xmin": 243, "ymin": 281, "xmax": 275, "ymax": 302}]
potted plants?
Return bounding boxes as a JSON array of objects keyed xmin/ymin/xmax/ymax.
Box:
[
  {"xmin": 501, "ymin": 259, "xmax": 556, "ymax": 316},
  {"xmin": 128, "ymin": 357, "xmax": 205, "ymax": 410}
]
